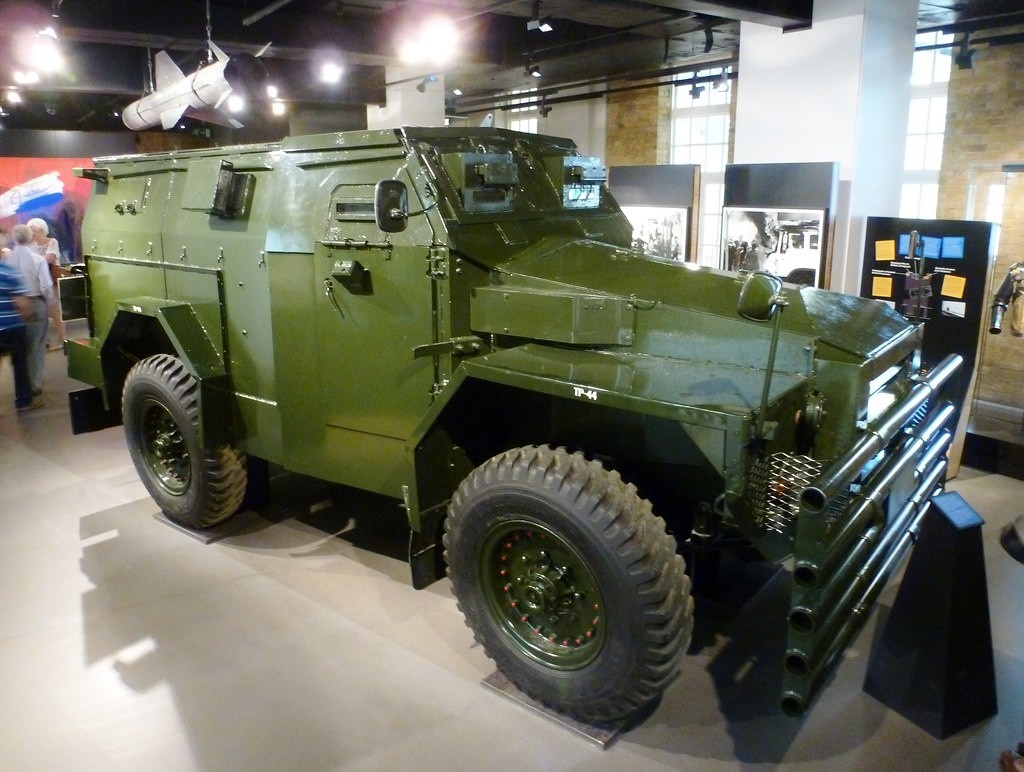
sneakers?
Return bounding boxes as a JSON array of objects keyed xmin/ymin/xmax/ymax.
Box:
[{"xmin": 15, "ymin": 395, "xmax": 49, "ymax": 412}]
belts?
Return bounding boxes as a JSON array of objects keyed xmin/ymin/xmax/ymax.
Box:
[{"xmin": 30, "ymin": 295, "xmax": 43, "ymax": 299}]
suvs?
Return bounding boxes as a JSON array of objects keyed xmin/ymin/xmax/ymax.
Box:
[{"xmin": 57, "ymin": 126, "xmax": 969, "ymax": 727}]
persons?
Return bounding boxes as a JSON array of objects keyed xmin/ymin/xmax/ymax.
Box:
[
  {"xmin": 0, "ymin": 248, "xmax": 46, "ymax": 412},
  {"xmin": 26, "ymin": 217, "xmax": 65, "ymax": 348},
  {"xmin": 3, "ymin": 223, "xmax": 64, "ymax": 398}
]
